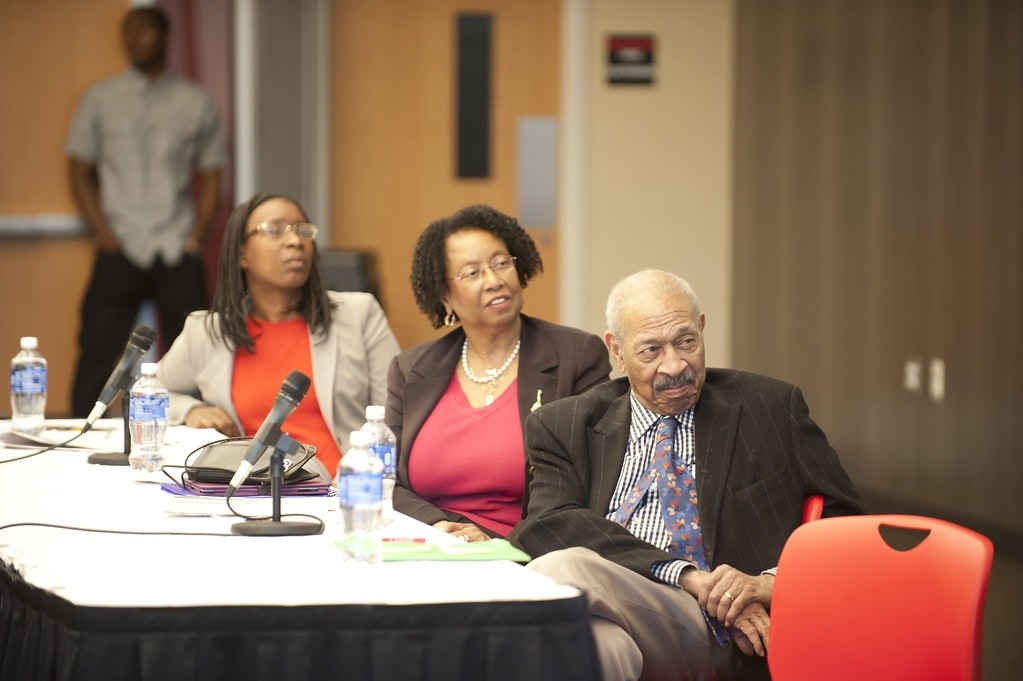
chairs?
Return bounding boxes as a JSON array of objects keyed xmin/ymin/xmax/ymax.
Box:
[{"xmin": 766, "ymin": 514, "xmax": 994, "ymax": 680}]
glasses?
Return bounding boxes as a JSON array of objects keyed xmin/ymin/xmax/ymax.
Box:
[
  {"xmin": 243, "ymin": 219, "xmax": 318, "ymax": 241},
  {"xmin": 444, "ymin": 255, "xmax": 517, "ymax": 288}
]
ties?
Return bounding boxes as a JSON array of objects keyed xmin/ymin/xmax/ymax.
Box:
[{"xmin": 611, "ymin": 416, "xmax": 732, "ymax": 648}]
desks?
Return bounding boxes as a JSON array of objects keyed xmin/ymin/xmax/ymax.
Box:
[{"xmin": 1, "ymin": 420, "xmax": 605, "ymax": 681}]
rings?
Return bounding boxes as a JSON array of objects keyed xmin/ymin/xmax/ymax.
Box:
[
  {"xmin": 724, "ymin": 592, "xmax": 735, "ymax": 601},
  {"xmin": 463, "ymin": 534, "xmax": 469, "ymax": 540}
]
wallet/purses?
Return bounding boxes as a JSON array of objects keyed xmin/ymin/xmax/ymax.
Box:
[{"xmin": 188, "ymin": 439, "xmax": 319, "ymax": 485}]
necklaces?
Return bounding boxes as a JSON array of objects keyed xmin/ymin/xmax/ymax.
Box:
[{"xmin": 462, "ymin": 338, "xmax": 521, "ymax": 407}]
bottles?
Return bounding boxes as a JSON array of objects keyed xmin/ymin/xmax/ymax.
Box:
[
  {"xmin": 362, "ymin": 404, "xmax": 397, "ymax": 527},
  {"xmin": 337, "ymin": 429, "xmax": 384, "ymax": 558},
  {"xmin": 10, "ymin": 336, "xmax": 47, "ymax": 438},
  {"xmin": 129, "ymin": 362, "xmax": 169, "ymax": 472}
]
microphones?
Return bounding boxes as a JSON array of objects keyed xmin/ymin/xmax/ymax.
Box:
[
  {"xmin": 226, "ymin": 370, "xmax": 311, "ymax": 498},
  {"xmin": 82, "ymin": 324, "xmax": 158, "ymax": 434}
]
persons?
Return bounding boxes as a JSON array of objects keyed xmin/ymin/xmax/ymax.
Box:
[
  {"xmin": 68, "ymin": 8, "xmax": 221, "ymax": 419},
  {"xmin": 383, "ymin": 203, "xmax": 613, "ymax": 542},
  {"xmin": 515, "ymin": 269, "xmax": 874, "ymax": 681},
  {"xmin": 154, "ymin": 191, "xmax": 402, "ymax": 478}
]
